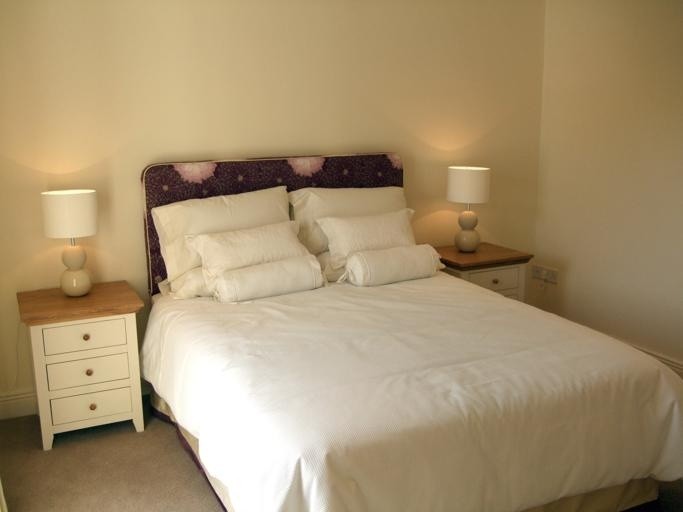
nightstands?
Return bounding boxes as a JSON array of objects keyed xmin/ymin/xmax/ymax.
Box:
[
  {"xmin": 436, "ymin": 241, "xmax": 531, "ymax": 302},
  {"xmin": 17, "ymin": 280, "xmax": 143, "ymax": 449}
]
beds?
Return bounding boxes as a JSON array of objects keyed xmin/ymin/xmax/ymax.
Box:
[{"xmin": 142, "ymin": 153, "xmax": 678, "ymax": 510}]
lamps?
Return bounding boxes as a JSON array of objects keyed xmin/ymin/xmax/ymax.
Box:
[
  {"xmin": 42, "ymin": 189, "xmax": 98, "ymax": 297},
  {"xmin": 445, "ymin": 168, "xmax": 489, "ymax": 253}
]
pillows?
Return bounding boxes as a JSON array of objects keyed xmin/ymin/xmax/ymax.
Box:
[{"xmin": 146, "ymin": 186, "xmax": 442, "ymax": 304}]
